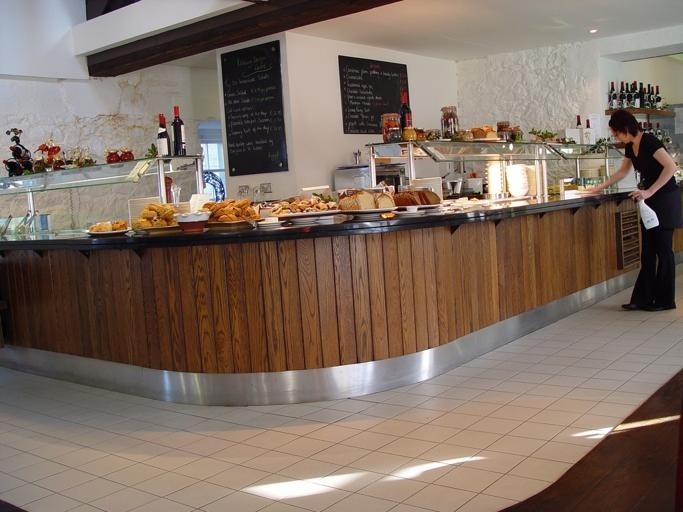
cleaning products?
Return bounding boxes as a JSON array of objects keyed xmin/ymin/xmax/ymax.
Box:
[{"xmin": 631, "ymin": 188, "xmax": 659, "ymax": 230}]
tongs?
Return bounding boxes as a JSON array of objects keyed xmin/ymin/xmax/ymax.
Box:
[
  {"xmin": 15, "ymin": 210, "xmax": 31, "ymax": 234},
  {"xmin": 18, "ymin": 210, "xmax": 40, "ymax": 234},
  {"xmin": 0, "ymin": 214, "xmax": 12, "ymax": 237}
]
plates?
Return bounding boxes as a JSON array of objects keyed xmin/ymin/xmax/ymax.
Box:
[
  {"xmin": 173, "ymin": 211, "xmax": 212, "ymax": 234},
  {"xmin": 208, "ymin": 218, "xmax": 265, "ymax": 232},
  {"xmin": 339, "ymin": 206, "xmax": 399, "ymax": 214},
  {"xmin": 141, "ymin": 225, "xmax": 179, "ymax": 235},
  {"xmin": 86, "ymin": 227, "xmax": 132, "ymax": 237},
  {"xmin": 397, "ymin": 204, "xmax": 440, "ymax": 212},
  {"xmin": 259, "ymin": 216, "xmax": 282, "ymax": 231},
  {"xmin": 276, "ymin": 209, "xmax": 341, "ymax": 221}
]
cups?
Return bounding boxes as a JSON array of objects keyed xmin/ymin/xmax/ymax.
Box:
[
  {"xmin": 353, "ymin": 152, "xmax": 362, "ymax": 166},
  {"xmin": 253, "ymin": 186, "xmax": 265, "ymax": 204},
  {"xmin": 239, "ymin": 185, "xmax": 249, "ymax": 201}
]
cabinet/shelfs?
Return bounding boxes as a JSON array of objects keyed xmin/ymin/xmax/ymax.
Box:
[
  {"xmin": 0, "ymin": 152, "xmax": 204, "ymax": 238},
  {"xmin": 600, "ymin": 103, "xmax": 678, "ymax": 155},
  {"xmin": 371, "ymin": 136, "xmax": 625, "ymax": 204}
]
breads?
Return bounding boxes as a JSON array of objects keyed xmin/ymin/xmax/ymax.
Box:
[
  {"xmin": 203, "ymin": 199, "xmax": 259, "ymax": 223},
  {"xmin": 392, "ymin": 188, "xmax": 441, "ymax": 205},
  {"xmin": 132, "ymin": 203, "xmax": 178, "ymax": 228},
  {"xmin": 88, "ymin": 219, "xmax": 126, "ymax": 232},
  {"xmin": 270, "ymin": 198, "xmax": 337, "ymax": 213},
  {"xmin": 472, "ymin": 126, "xmax": 496, "ymax": 137},
  {"xmin": 338, "ymin": 192, "xmax": 396, "ymax": 210}
]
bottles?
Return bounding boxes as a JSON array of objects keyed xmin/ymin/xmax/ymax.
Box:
[
  {"xmin": 157, "ymin": 113, "xmax": 171, "ymax": 158},
  {"xmin": 656, "ymin": 122, "xmax": 661, "ymax": 138},
  {"xmin": 647, "ymin": 84, "xmax": 651, "ymax": 103},
  {"xmin": 586, "ymin": 119, "xmax": 592, "ymax": 132},
  {"xmin": 651, "ymin": 86, "xmax": 655, "ymax": 104},
  {"xmin": 649, "ymin": 122, "xmax": 655, "ymax": 136},
  {"xmin": 400, "ymin": 87, "xmax": 412, "ymax": 127},
  {"xmin": 171, "ymin": 105, "xmax": 186, "ymax": 156},
  {"xmin": 625, "ymin": 82, "xmax": 630, "ymax": 95},
  {"xmin": 10, "ymin": 146, "xmax": 30, "ymax": 157},
  {"xmin": 608, "ymin": 81, "xmax": 618, "ymax": 108},
  {"xmin": 19, "ymin": 210, "xmax": 39, "ymax": 237},
  {"xmin": 13, "ymin": 210, "xmax": 31, "ymax": 236},
  {"xmin": 638, "ymin": 82, "xmax": 644, "ymax": 108},
  {"xmin": 656, "ymin": 86, "xmax": 661, "ymax": 109},
  {"xmin": 3, "ymin": 159, "xmax": 24, "ymax": 177},
  {"xmin": 644, "ymin": 122, "xmax": 649, "ymax": 131},
  {"xmin": 639, "ymin": 121, "xmax": 644, "ymax": 129},
  {"xmin": 631, "ymin": 83, "xmax": 634, "ymax": 93},
  {"xmin": 0, "ymin": 214, "xmax": 13, "ymax": 236},
  {"xmin": 643, "ymin": 87, "xmax": 648, "ymax": 103},
  {"xmin": 633, "ymin": 79, "xmax": 640, "ymax": 109},
  {"xmin": 629, "ymin": 83, "xmax": 632, "ymax": 95},
  {"xmin": 576, "ymin": 115, "xmax": 582, "ymax": 129},
  {"xmin": 619, "ymin": 81, "xmax": 626, "ymax": 108}
]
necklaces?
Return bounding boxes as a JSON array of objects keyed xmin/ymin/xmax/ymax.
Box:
[{"xmin": 633, "ymin": 133, "xmax": 642, "ymax": 157}]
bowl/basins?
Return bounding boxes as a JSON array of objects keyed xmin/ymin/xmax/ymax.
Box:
[{"xmin": 506, "ymin": 163, "xmax": 529, "ymax": 197}]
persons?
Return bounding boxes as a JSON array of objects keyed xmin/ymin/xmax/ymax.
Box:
[{"xmin": 586, "ymin": 109, "xmax": 683, "ymax": 311}]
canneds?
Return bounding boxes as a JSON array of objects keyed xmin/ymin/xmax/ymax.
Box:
[
  {"xmin": 496, "ymin": 122, "xmax": 513, "ymax": 143},
  {"xmin": 414, "ymin": 128, "xmax": 426, "ymax": 140},
  {"xmin": 387, "ymin": 127, "xmax": 401, "ymax": 142},
  {"xmin": 404, "ymin": 126, "xmax": 416, "ymax": 141},
  {"xmin": 511, "ymin": 127, "xmax": 522, "ymax": 144},
  {"xmin": 441, "ymin": 106, "xmax": 459, "ymax": 138},
  {"xmin": 381, "ymin": 113, "xmax": 402, "ymax": 142}
]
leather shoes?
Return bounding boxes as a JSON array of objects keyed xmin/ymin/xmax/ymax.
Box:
[
  {"xmin": 622, "ymin": 302, "xmax": 652, "ymax": 310},
  {"xmin": 647, "ymin": 304, "xmax": 676, "ymax": 312}
]
